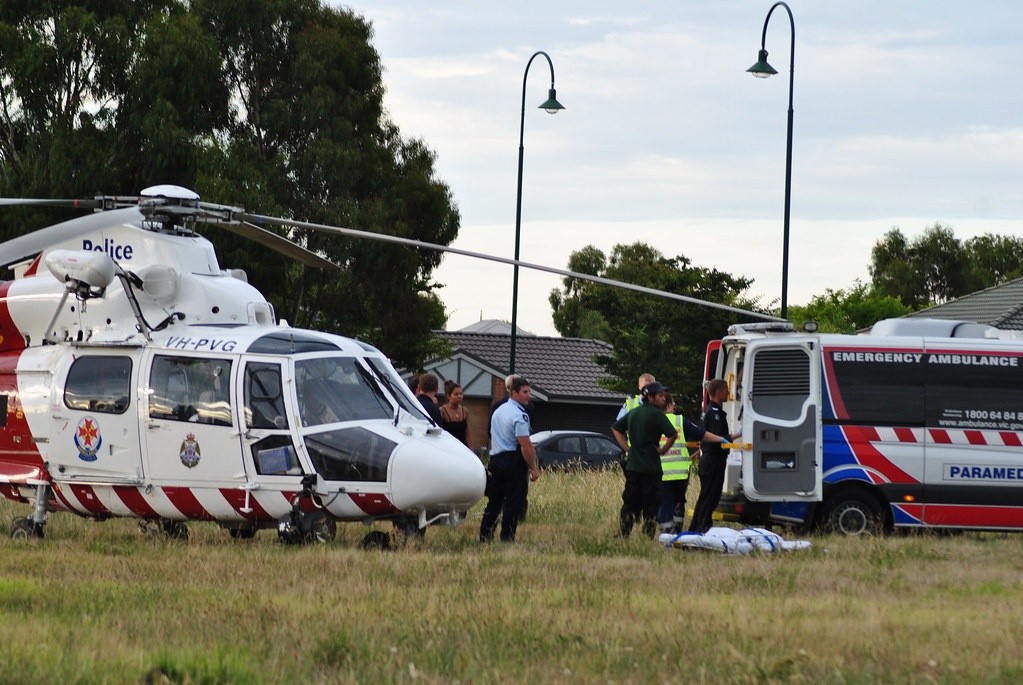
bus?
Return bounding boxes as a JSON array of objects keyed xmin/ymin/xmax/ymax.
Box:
[{"xmin": 700, "ymin": 314, "xmax": 1023, "ymax": 538}]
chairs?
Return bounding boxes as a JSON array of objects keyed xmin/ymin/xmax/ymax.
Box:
[
  {"xmin": 162, "ymin": 371, "xmax": 193, "ymax": 419},
  {"xmin": 245, "ymin": 368, "xmax": 289, "ymax": 431},
  {"xmin": 566, "ymin": 443, "xmax": 577, "ymax": 453}
]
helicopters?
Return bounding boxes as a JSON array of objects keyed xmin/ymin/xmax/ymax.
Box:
[{"xmin": 0, "ymin": 186, "xmax": 486, "ymax": 548}]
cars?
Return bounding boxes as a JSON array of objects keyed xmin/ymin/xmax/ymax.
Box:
[{"xmin": 530, "ymin": 429, "xmax": 623, "ymax": 472}]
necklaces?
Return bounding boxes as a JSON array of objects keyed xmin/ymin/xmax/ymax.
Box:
[{"xmin": 455, "ymin": 413, "xmax": 459, "ymax": 419}]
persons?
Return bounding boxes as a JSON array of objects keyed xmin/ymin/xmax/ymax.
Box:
[
  {"xmin": 479, "ymin": 374, "xmax": 540, "ymax": 543},
  {"xmin": 687, "ymin": 378, "xmax": 742, "ymax": 535},
  {"xmin": 611, "ymin": 373, "xmax": 730, "ymax": 539},
  {"xmin": 408, "ymin": 373, "xmax": 474, "ymax": 521}
]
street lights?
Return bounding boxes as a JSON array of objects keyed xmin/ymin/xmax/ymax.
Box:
[
  {"xmin": 507, "ymin": 51, "xmax": 565, "ymax": 377},
  {"xmin": 745, "ymin": 1, "xmax": 796, "ymax": 320}
]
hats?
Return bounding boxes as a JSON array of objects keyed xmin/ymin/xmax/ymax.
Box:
[{"xmin": 641, "ymin": 382, "xmax": 668, "ymax": 395}]
